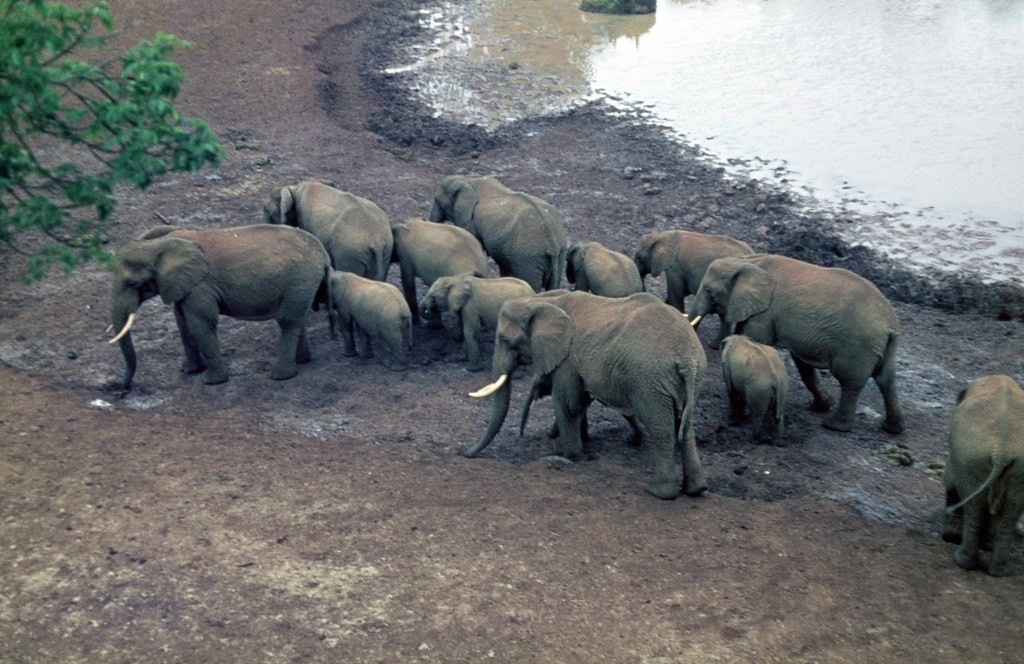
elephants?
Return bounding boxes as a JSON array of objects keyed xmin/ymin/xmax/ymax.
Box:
[
  {"xmin": 940, "ymin": 371, "xmax": 1024, "ymax": 578},
  {"xmin": 99, "ymin": 170, "xmax": 909, "ymax": 579}
]
